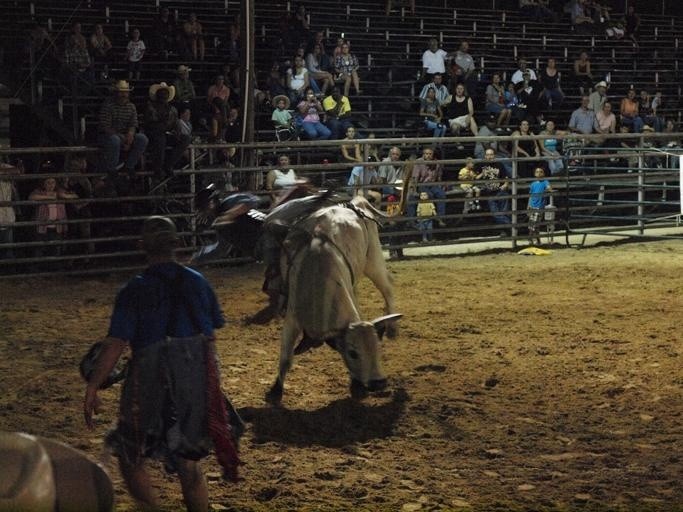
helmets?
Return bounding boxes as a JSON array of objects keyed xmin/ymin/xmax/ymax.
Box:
[{"xmin": 194, "ymin": 189, "xmax": 220, "ymax": 208}]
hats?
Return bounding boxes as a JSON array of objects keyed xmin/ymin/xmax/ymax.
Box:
[
  {"xmin": 0, "ymin": 430, "xmax": 115, "ymax": 512},
  {"xmin": 114, "ymin": 80, "xmax": 134, "ymax": 92},
  {"xmin": 595, "ymin": 81, "xmax": 607, "ymax": 89},
  {"xmin": 272, "ymin": 95, "xmax": 290, "ymax": 110},
  {"xmin": 177, "ymin": 65, "xmax": 191, "ymax": 73},
  {"xmin": 149, "ymin": 82, "xmax": 176, "ymax": 102},
  {"xmin": 640, "ymin": 126, "xmax": 655, "ymax": 132}
]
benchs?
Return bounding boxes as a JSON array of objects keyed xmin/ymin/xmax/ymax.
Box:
[{"xmin": 0, "ymin": 1, "xmax": 682, "ymax": 210}]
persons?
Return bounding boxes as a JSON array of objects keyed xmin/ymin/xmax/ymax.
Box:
[
  {"xmin": 82, "ymin": 216, "xmax": 226, "ymax": 512},
  {"xmin": 1, "ymin": 1, "xmax": 683, "ymax": 327}
]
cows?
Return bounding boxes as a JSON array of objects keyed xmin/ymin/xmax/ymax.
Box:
[{"xmin": 265, "ymin": 161, "xmax": 415, "ymax": 394}]
